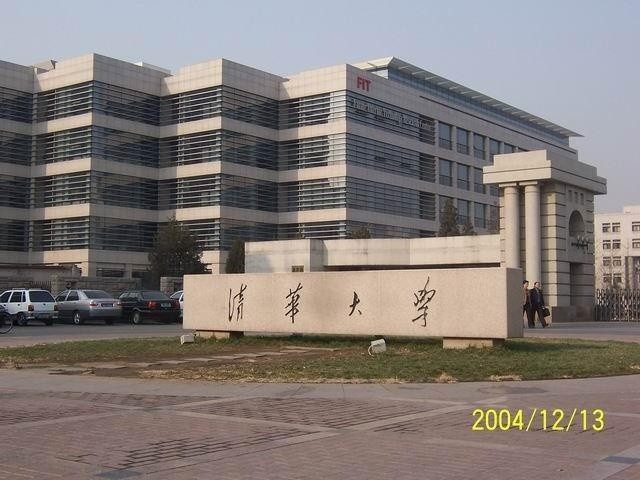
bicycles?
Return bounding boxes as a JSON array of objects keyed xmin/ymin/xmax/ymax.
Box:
[{"xmin": 0, "ymin": 310, "xmax": 16, "ymax": 338}]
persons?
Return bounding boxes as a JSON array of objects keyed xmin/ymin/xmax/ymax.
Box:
[
  {"xmin": 530, "ymin": 282, "xmax": 549, "ymax": 328},
  {"xmin": 523, "ymin": 280, "xmax": 535, "ymax": 328}
]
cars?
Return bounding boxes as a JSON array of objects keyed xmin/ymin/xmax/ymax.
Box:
[
  {"xmin": 50, "ymin": 288, "xmax": 124, "ymax": 327},
  {"xmin": 113, "ymin": 289, "xmax": 180, "ymax": 326},
  {"xmin": 170, "ymin": 290, "xmax": 184, "ymax": 319}
]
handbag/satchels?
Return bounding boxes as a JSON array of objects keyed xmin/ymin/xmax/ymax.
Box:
[{"xmin": 540, "ymin": 306, "xmax": 551, "ymax": 316}]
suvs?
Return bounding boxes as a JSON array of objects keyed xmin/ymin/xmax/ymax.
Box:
[{"xmin": 0, "ymin": 285, "xmax": 61, "ymax": 328}]
